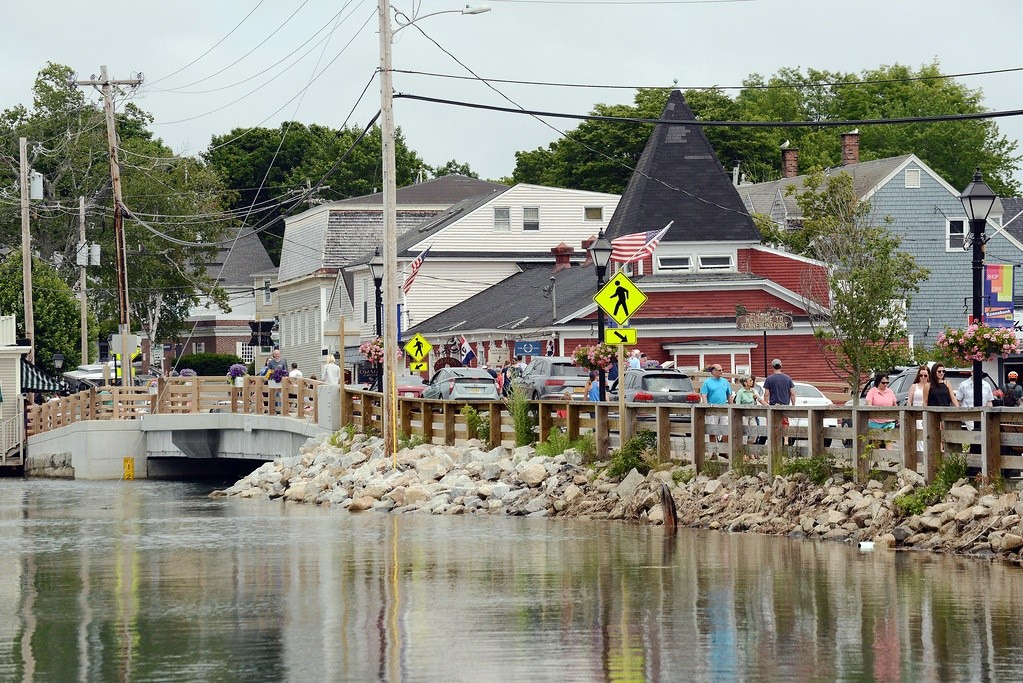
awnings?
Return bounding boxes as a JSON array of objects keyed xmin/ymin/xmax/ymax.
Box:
[{"xmin": 21, "ymin": 358, "xmax": 65, "ymax": 393}]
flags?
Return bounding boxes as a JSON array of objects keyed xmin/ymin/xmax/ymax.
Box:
[
  {"xmin": 610, "ymin": 227, "xmax": 667, "ymax": 262},
  {"xmin": 403, "ymin": 246, "xmax": 431, "ymax": 295},
  {"xmin": 461, "ymin": 337, "xmax": 476, "ymax": 367}
]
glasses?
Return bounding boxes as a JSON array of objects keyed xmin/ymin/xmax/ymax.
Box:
[
  {"xmin": 715, "ymin": 369, "xmax": 723, "ymax": 372},
  {"xmin": 938, "ymin": 370, "xmax": 945, "ymax": 373},
  {"xmin": 919, "ymin": 374, "xmax": 928, "ymax": 377},
  {"xmin": 880, "ymin": 381, "xmax": 889, "ymax": 384}
]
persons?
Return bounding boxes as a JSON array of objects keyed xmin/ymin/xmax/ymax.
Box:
[
  {"xmin": 626, "ymin": 349, "xmax": 659, "ymax": 369},
  {"xmin": 734, "ymin": 374, "xmax": 769, "ymax": 461},
  {"xmin": 262, "ymin": 349, "xmax": 340, "ymax": 414},
  {"xmin": 923, "ymin": 363, "xmax": 959, "ymax": 407},
  {"xmin": 1001, "ymin": 371, "xmax": 1023, "ymax": 407},
  {"xmin": 557, "ymin": 370, "xmax": 610, "ymax": 437},
  {"xmin": 867, "ymin": 373, "xmax": 899, "ymax": 467},
  {"xmin": 955, "ymin": 365, "xmax": 994, "ymax": 432},
  {"xmin": 763, "ymin": 359, "xmax": 795, "ymax": 447},
  {"xmin": 482, "ymin": 354, "xmax": 527, "ymax": 397},
  {"xmin": 700, "ymin": 363, "xmax": 733, "ymax": 460},
  {"xmin": 907, "ymin": 365, "xmax": 930, "ymax": 451}
]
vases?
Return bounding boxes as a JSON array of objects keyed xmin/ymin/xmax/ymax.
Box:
[
  {"xmin": 184, "ymin": 381, "xmax": 192, "ymax": 386},
  {"xmin": 268, "ymin": 379, "xmax": 282, "ymax": 388},
  {"xmin": 149, "ymin": 387, "xmax": 157, "ymax": 395},
  {"xmin": 231, "ymin": 376, "xmax": 244, "ymax": 387}
]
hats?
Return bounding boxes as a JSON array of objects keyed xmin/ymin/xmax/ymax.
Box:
[{"xmin": 772, "ymin": 359, "xmax": 782, "ymax": 367}]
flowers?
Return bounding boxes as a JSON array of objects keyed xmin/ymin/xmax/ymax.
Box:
[
  {"xmin": 265, "ymin": 369, "xmax": 289, "ymax": 383},
  {"xmin": 936, "ymin": 319, "xmax": 1021, "ymax": 367},
  {"xmin": 226, "ymin": 364, "xmax": 249, "ymax": 385},
  {"xmin": 178, "ymin": 369, "xmax": 197, "ymax": 385},
  {"xmin": 360, "ymin": 337, "xmax": 403, "ymax": 363},
  {"xmin": 571, "ymin": 342, "xmax": 632, "ymax": 377},
  {"xmin": 146, "ymin": 380, "xmax": 157, "ymax": 391}
]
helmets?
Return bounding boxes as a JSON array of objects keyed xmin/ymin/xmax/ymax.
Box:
[{"xmin": 1008, "ymin": 371, "xmax": 1018, "ymax": 378}]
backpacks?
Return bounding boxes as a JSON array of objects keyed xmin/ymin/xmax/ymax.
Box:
[{"xmin": 1004, "ymin": 384, "xmax": 1018, "ymax": 406}]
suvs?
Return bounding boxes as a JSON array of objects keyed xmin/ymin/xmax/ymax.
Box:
[
  {"xmin": 63, "ymin": 361, "xmax": 167, "ymax": 393},
  {"xmin": 840, "ymin": 364, "xmax": 1005, "ymax": 450},
  {"xmin": 604, "ymin": 367, "xmax": 701, "ymax": 436}
]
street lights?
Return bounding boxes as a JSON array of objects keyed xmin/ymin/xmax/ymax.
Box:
[
  {"xmin": 367, "ymin": 246, "xmax": 384, "ymax": 393},
  {"xmin": 958, "ymin": 165, "xmax": 1000, "ymax": 475},
  {"xmin": 377, "ymin": 5, "xmax": 492, "ymax": 460}
]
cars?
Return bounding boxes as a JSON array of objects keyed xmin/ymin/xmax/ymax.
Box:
[
  {"xmin": 420, "ymin": 366, "xmax": 500, "ymax": 413},
  {"xmin": 716, "ymin": 382, "xmax": 838, "ymax": 447},
  {"xmin": 368, "ymin": 370, "xmax": 429, "ymax": 411},
  {"xmin": 508, "ymin": 356, "xmax": 609, "ymax": 421}
]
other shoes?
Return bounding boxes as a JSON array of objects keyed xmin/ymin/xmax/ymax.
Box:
[
  {"xmin": 710, "ymin": 455, "xmax": 718, "ymax": 460},
  {"xmin": 888, "ymin": 462, "xmax": 899, "ymax": 467},
  {"xmin": 872, "ymin": 462, "xmax": 882, "ymax": 468},
  {"xmin": 719, "ymin": 453, "xmax": 728, "ymax": 458}
]
